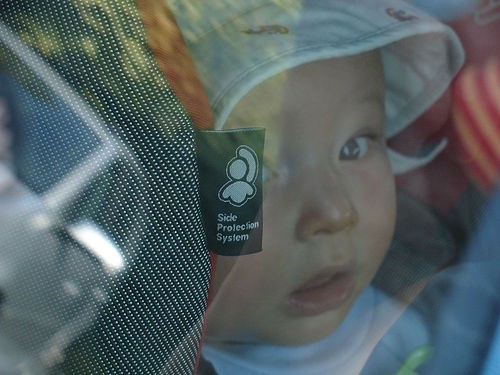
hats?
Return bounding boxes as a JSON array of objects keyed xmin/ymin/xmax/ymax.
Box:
[{"xmin": 172, "ymin": 0, "xmax": 467, "ymax": 142}]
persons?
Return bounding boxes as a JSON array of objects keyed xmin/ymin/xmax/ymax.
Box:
[{"xmin": 164, "ymin": 0, "xmax": 500, "ymax": 374}]
